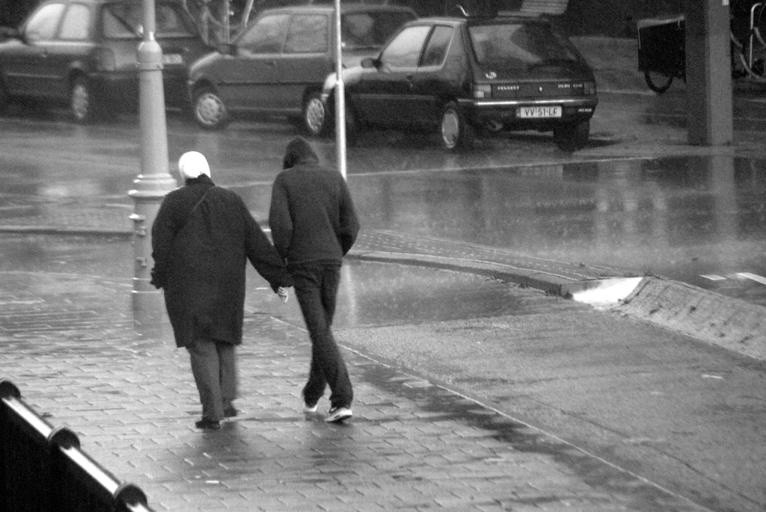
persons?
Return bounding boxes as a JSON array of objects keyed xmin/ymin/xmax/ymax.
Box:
[
  {"xmin": 151, "ymin": 151, "xmax": 294, "ymax": 429},
  {"xmin": 270, "ymin": 136, "xmax": 360, "ymax": 423}
]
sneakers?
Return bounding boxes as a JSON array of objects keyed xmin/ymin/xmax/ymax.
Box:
[
  {"xmin": 323, "ymin": 403, "xmax": 353, "ymax": 423},
  {"xmin": 297, "ymin": 383, "xmax": 319, "ymax": 413},
  {"xmin": 194, "ymin": 404, "xmax": 239, "ymax": 429}
]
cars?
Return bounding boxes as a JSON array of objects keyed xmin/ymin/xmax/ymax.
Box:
[
  {"xmin": 319, "ymin": 11, "xmax": 601, "ymax": 151},
  {"xmin": 187, "ymin": 2, "xmax": 420, "ymax": 129},
  {"xmin": 0, "ymin": 0, "xmax": 218, "ymax": 123}
]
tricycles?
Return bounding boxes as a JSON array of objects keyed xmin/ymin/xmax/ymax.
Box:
[{"xmin": 637, "ymin": 0, "xmax": 765, "ymax": 95}]
